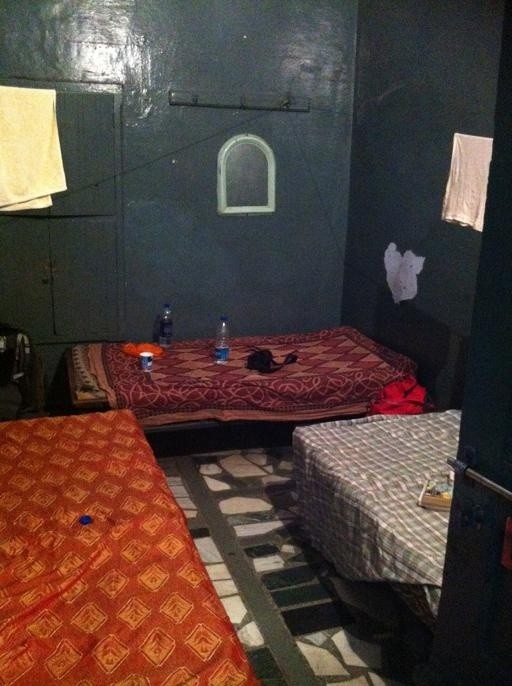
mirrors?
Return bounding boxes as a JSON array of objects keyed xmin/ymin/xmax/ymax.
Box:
[{"xmin": 210, "ymin": 130, "xmax": 279, "ymax": 218}]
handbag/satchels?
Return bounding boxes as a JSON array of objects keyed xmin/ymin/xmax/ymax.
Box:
[{"xmin": 246, "ymin": 348, "xmax": 299, "ymax": 374}]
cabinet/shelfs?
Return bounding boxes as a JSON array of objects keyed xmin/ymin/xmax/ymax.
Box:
[
  {"xmin": 0, "ymin": 88, "xmax": 127, "ymax": 218},
  {"xmin": 1, "ymin": 215, "xmax": 129, "ymax": 347},
  {"xmin": 29, "ymin": 340, "xmax": 79, "ymax": 418}
]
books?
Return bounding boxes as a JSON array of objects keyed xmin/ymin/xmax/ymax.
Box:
[{"xmin": 418, "ymin": 474, "xmax": 455, "ymax": 511}]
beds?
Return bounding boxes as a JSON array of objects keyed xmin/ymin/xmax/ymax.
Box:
[
  {"xmin": 57, "ymin": 318, "xmax": 425, "ymax": 435},
  {"xmin": 285, "ymin": 401, "xmax": 470, "ymax": 638},
  {"xmin": 1, "ymin": 405, "xmax": 263, "ymax": 686}
]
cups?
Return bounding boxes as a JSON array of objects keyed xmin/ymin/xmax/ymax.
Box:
[{"xmin": 140, "ymin": 352, "xmax": 154, "ymax": 372}]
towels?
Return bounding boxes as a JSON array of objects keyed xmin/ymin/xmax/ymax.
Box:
[
  {"xmin": 433, "ymin": 125, "xmax": 496, "ymax": 236},
  {"xmin": 0, "ymin": 82, "xmax": 70, "ymax": 216}
]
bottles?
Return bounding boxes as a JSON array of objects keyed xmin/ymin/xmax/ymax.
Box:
[
  {"xmin": 158, "ymin": 303, "xmax": 175, "ymax": 348},
  {"xmin": 215, "ymin": 316, "xmax": 231, "ymax": 363}
]
